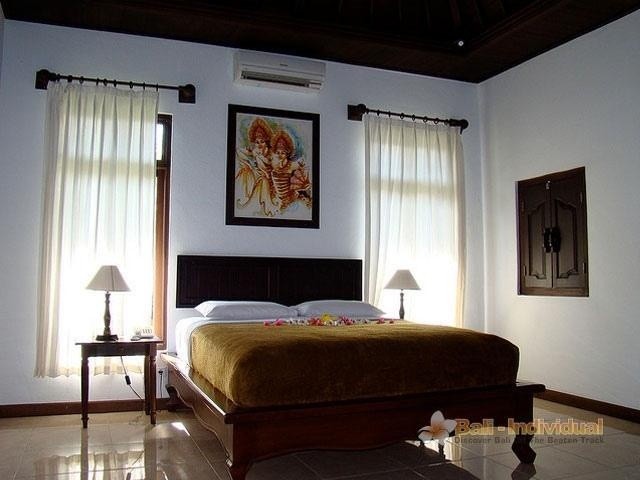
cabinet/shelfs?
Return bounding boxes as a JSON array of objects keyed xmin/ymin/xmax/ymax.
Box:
[{"xmin": 516, "ymin": 167, "xmax": 586, "ymax": 296}]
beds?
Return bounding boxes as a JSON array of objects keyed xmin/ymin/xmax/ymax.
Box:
[
  {"xmin": 75, "ymin": 339, "xmax": 161, "ymax": 424},
  {"xmin": 159, "ymin": 309, "xmax": 547, "ymax": 480}
]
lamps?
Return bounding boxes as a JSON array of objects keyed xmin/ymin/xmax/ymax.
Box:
[
  {"xmin": 85, "ymin": 264, "xmax": 131, "ymax": 341},
  {"xmin": 386, "ymin": 269, "xmax": 422, "ymax": 318}
]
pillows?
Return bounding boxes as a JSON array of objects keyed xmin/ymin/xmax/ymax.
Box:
[{"xmin": 196, "ymin": 300, "xmax": 384, "ymax": 317}]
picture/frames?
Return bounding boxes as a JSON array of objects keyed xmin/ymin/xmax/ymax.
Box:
[{"xmin": 228, "ymin": 104, "xmax": 319, "ymax": 229}]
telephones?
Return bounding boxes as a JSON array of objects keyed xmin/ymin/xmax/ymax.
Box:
[{"xmin": 134, "ymin": 327, "xmax": 153, "ymax": 338}]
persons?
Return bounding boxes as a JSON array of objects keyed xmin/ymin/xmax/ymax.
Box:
[{"xmin": 237, "ymin": 117, "xmax": 311, "ymax": 206}]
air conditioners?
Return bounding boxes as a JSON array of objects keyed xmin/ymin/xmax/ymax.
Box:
[{"xmin": 229, "ymin": 50, "xmax": 326, "ymax": 95}]
